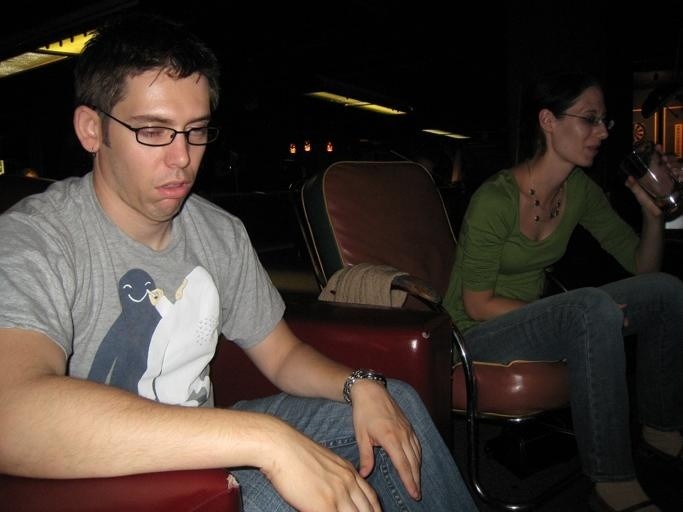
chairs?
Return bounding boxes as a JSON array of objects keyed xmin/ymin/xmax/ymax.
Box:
[
  {"xmin": 0, "ymin": 299, "xmax": 452, "ymax": 511},
  {"xmin": 297, "ymin": 161, "xmax": 574, "ymax": 511}
]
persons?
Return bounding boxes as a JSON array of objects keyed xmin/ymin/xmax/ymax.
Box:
[
  {"xmin": 0, "ymin": 17, "xmax": 478, "ymax": 512},
  {"xmin": 442, "ymin": 65, "xmax": 683, "ymax": 512}
]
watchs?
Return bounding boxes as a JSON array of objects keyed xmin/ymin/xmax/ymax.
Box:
[{"xmin": 343, "ymin": 367, "xmax": 387, "ymax": 404}]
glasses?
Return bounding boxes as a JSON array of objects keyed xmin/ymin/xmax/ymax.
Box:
[
  {"xmin": 555, "ymin": 113, "xmax": 614, "ymax": 130},
  {"xmin": 84, "ymin": 103, "xmax": 220, "ymax": 146}
]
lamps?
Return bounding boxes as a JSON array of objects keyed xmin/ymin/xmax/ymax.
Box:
[
  {"xmin": 421, "ymin": 125, "xmax": 471, "ymax": 140},
  {"xmin": 0, "ymin": 1, "xmax": 138, "ymax": 81},
  {"xmin": 302, "ymin": 75, "xmax": 412, "ymax": 118}
]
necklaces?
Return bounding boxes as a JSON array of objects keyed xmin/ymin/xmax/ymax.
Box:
[{"xmin": 526, "ymin": 161, "xmax": 565, "ymax": 223}]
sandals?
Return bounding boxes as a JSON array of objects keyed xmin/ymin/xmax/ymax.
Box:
[
  {"xmin": 589, "ymin": 475, "xmax": 661, "ymax": 512},
  {"xmin": 636, "ymin": 424, "xmax": 683, "ymax": 464}
]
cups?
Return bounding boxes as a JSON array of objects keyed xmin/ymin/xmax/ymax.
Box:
[{"xmin": 623, "ymin": 138, "xmax": 682, "ymax": 222}]
